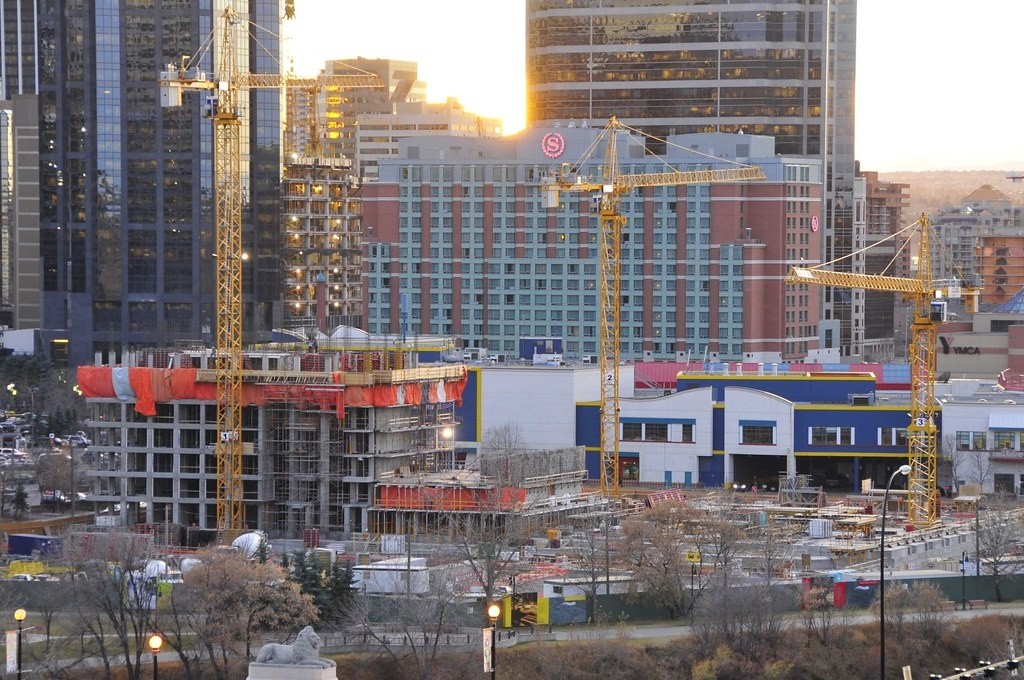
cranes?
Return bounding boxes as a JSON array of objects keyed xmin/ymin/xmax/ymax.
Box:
[
  {"xmin": 539, "ymin": 116, "xmax": 766, "ymax": 499},
  {"xmin": 159, "ymin": 6, "xmax": 385, "ymax": 546},
  {"xmin": 784, "ymin": 212, "xmax": 984, "ymax": 527}
]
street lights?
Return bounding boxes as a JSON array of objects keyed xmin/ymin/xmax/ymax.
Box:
[
  {"xmin": 881, "ymin": 465, "xmax": 912, "ymax": 680},
  {"xmin": 961, "ymin": 550, "xmax": 969, "ymax": 610},
  {"xmin": 155, "ymin": 584, "xmax": 161, "ymax": 628},
  {"xmin": 691, "ymin": 564, "xmax": 696, "ymax": 597},
  {"xmin": 510, "ymin": 574, "xmax": 515, "ymax": 627},
  {"xmin": 14, "ymin": 609, "xmax": 26, "ymax": 680},
  {"xmin": 488, "ymin": 604, "xmax": 501, "ymax": 680},
  {"xmin": 149, "ymin": 636, "xmax": 163, "ymax": 680}
]
